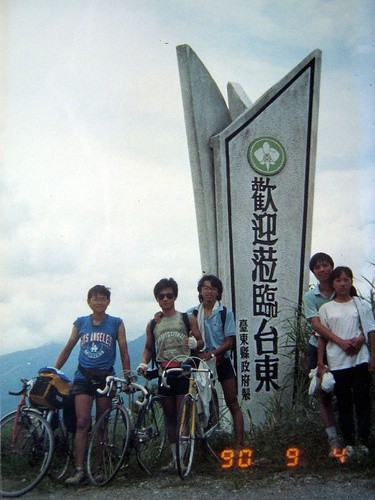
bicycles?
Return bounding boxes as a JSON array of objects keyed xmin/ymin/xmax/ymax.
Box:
[
  {"xmin": 86, "ymin": 374, "xmax": 169, "ymax": 489},
  {"xmin": 160, "ymin": 366, "xmax": 224, "ymax": 482},
  {"xmin": 0, "ymin": 375, "xmax": 79, "ymax": 496}
]
